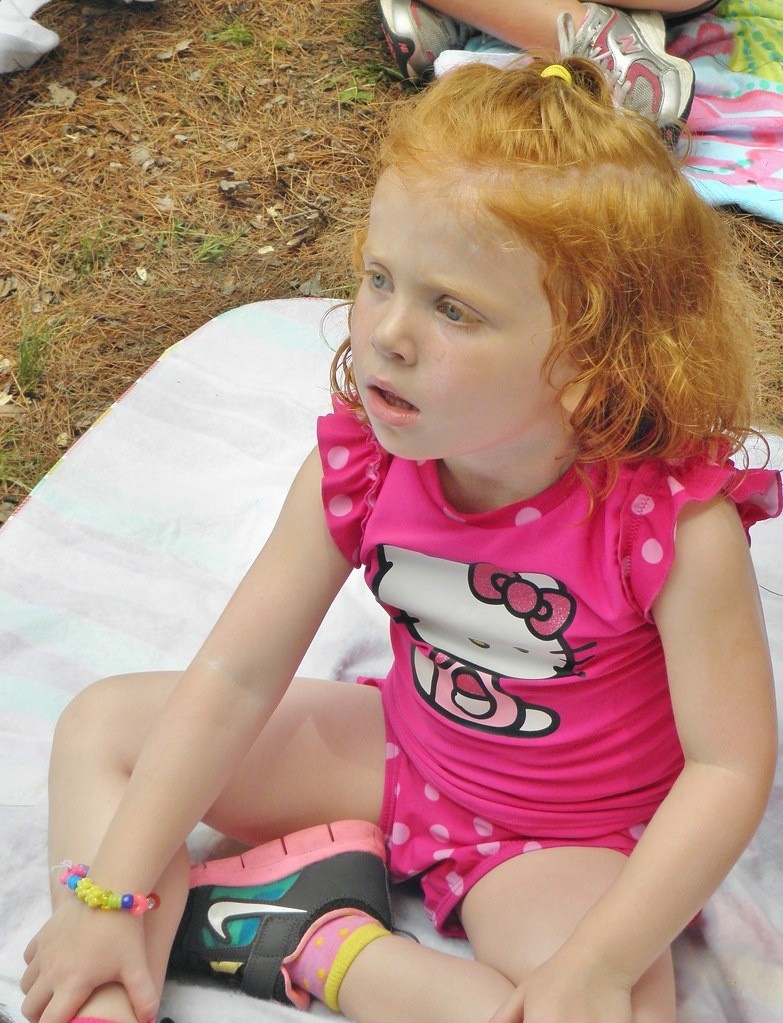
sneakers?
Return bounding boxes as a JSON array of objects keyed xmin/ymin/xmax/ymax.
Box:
[
  {"xmin": 378, "ymin": 0, "xmax": 486, "ymax": 85},
  {"xmin": 557, "ymin": 1, "xmax": 696, "ymax": 131},
  {"xmin": 165, "ymin": 818, "xmax": 396, "ymax": 1012}
]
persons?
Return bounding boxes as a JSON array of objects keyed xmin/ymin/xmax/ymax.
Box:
[
  {"xmin": 17, "ymin": 54, "xmax": 778, "ymax": 1023},
  {"xmin": 378, "ymin": 0, "xmax": 721, "ymax": 152}
]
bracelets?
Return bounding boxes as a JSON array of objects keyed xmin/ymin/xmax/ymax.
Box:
[{"xmin": 61, "ymin": 862, "xmax": 162, "ymax": 914}]
